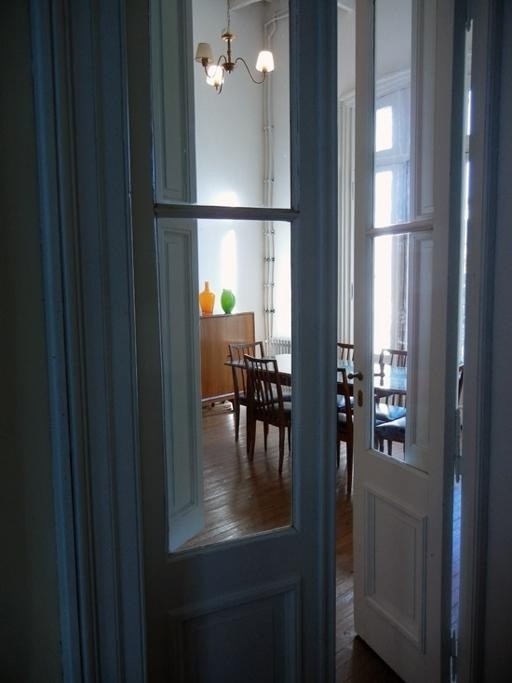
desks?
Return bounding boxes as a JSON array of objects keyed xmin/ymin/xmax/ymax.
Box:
[{"xmin": 196, "ymin": 311, "xmax": 256, "ymax": 412}]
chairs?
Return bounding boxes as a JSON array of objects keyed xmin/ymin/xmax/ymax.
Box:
[{"xmin": 225, "ymin": 340, "xmax": 465, "ymax": 496}]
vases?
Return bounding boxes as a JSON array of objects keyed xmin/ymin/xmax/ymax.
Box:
[
  {"xmin": 198, "ymin": 281, "xmax": 216, "ymax": 317},
  {"xmin": 221, "ymin": 288, "xmax": 235, "ymax": 314}
]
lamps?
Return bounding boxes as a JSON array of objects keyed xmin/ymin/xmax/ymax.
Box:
[{"xmin": 195, "ymin": 0, "xmax": 275, "ymax": 95}]
